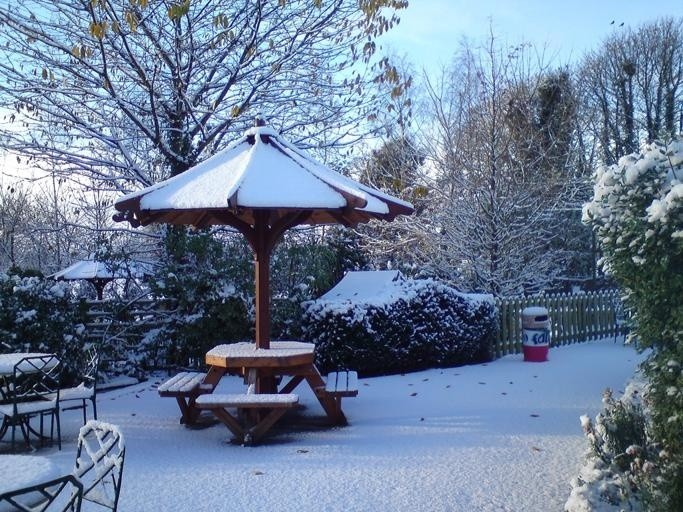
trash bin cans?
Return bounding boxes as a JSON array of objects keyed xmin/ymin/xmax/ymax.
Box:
[{"xmin": 521, "ymin": 306, "xmax": 551, "ymax": 362}]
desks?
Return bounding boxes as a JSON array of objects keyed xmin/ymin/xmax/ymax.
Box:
[
  {"xmin": 0, "ymin": 452, "xmax": 63, "ymax": 512},
  {"xmin": 180, "ymin": 340, "xmax": 350, "ymax": 432}
]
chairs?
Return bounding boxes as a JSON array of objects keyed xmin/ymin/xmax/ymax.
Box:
[
  {"xmin": 621, "ymin": 305, "xmax": 639, "ymax": 348},
  {"xmin": 0, "ymin": 473, "xmax": 84, "ymax": 512},
  {"xmin": 610, "ymin": 297, "xmax": 638, "ymax": 348},
  {"xmin": 35, "ymin": 340, "xmax": 102, "ymax": 451},
  {"xmin": 68, "ymin": 418, "xmax": 125, "ymax": 512}
]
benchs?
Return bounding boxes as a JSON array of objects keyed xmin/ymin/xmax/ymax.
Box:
[
  {"xmin": 322, "ymin": 370, "xmax": 359, "ymax": 427},
  {"xmin": 156, "ymin": 370, "xmax": 209, "ymax": 430},
  {"xmin": 193, "ymin": 390, "xmax": 306, "ymax": 447}
]
